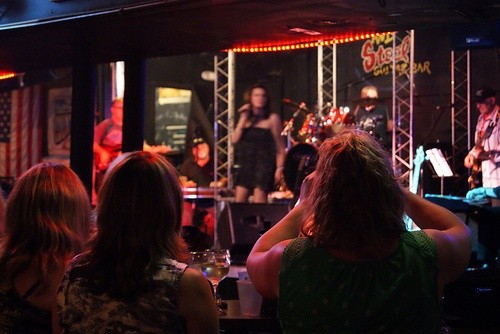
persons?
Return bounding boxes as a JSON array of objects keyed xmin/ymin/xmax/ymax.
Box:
[
  {"xmin": 463, "ymin": 89, "xmax": 500, "ymax": 207},
  {"xmin": 51, "ymin": 151, "xmax": 220, "ymax": 334},
  {"xmin": 179, "ymin": 141, "xmax": 214, "ymax": 189},
  {"xmin": 230, "ymin": 85, "xmax": 288, "ymax": 203},
  {"xmin": 354, "ymin": 86, "xmax": 391, "ymax": 161},
  {"xmin": 94, "ymin": 100, "xmax": 123, "ymax": 201},
  {"xmin": 0, "ymin": 163, "xmax": 98, "ymax": 334},
  {"xmin": 246, "ymin": 130, "xmax": 473, "ymax": 334}
]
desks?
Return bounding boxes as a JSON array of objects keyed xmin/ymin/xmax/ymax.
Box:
[{"xmin": 209, "ymin": 265, "xmax": 279, "ymax": 334}]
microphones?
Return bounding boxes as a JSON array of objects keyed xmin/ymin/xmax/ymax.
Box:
[
  {"xmin": 238, "ymin": 103, "xmax": 253, "ymax": 112},
  {"xmin": 436, "ymin": 102, "xmax": 456, "ymax": 111}
]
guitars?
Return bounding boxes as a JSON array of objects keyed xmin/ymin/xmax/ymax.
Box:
[
  {"xmin": 402, "ymin": 146, "xmax": 424, "ymax": 232},
  {"xmin": 470, "ymin": 145, "xmax": 500, "ymax": 175}
]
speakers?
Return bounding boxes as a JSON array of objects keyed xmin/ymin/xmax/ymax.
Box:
[{"xmin": 215, "ymin": 201, "xmax": 290, "ymax": 263}]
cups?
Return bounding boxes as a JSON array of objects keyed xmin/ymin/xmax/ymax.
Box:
[{"xmin": 237, "ymin": 281, "xmax": 261, "ymax": 316}]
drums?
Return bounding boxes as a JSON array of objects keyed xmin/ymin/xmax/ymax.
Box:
[{"xmin": 280, "ymin": 142, "xmax": 320, "ymax": 195}]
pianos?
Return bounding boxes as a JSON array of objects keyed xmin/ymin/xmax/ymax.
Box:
[{"xmin": 183, "ymin": 186, "xmax": 228, "ymax": 201}]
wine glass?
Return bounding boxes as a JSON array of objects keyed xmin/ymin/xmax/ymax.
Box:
[
  {"xmin": 204, "ymin": 249, "xmax": 230, "ymax": 307},
  {"xmin": 202, "ymin": 254, "xmax": 231, "ymax": 316}
]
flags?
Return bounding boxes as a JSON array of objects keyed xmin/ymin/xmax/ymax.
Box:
[{"xmin": 0, "ymin": 83, "xmax": 42, "ymax": 177}]
